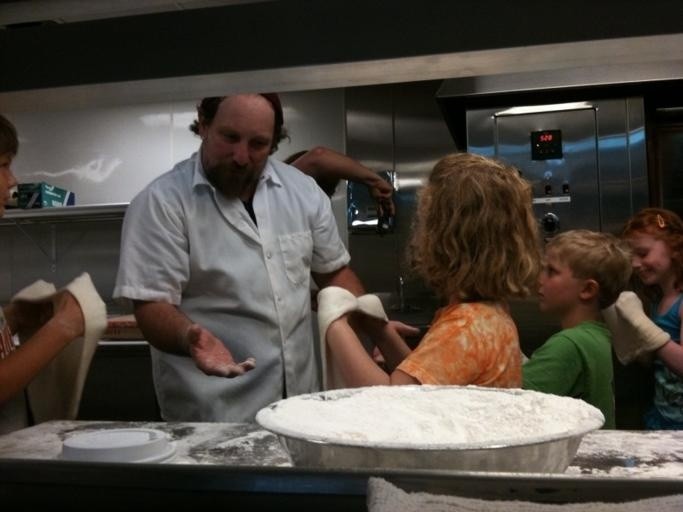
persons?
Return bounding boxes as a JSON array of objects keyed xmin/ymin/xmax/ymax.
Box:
[
  {"xmin": 322, "ymin": 152, "xmax": 551, "ymax": 389},
  {"xmin": 614, "ymin": 206, "xmax": 683, "ymax": 432},
  {"xmin": 110, "ymin": 90, "xmax": 423, "ymax": 424},
  {"xmin": 0, "ymin": 116, "xmax": 86, "ymax": 438},
  {"xmin": 280, "ymin": 146, "xmax": 398, "ymax": 222},
  {"xmin": 522, "ymin": 228, "xmax": 634, "ymax": 429}
]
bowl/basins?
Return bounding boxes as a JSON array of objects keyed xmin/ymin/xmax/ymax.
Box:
[{"xmin": 252, "ymin": 382, "xmax": 607, "ymax": 474}]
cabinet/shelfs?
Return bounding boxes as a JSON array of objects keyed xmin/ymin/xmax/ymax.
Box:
[{"xmin": 1, "ymin": 202, "xmax": 152, "ymax": 354}]
